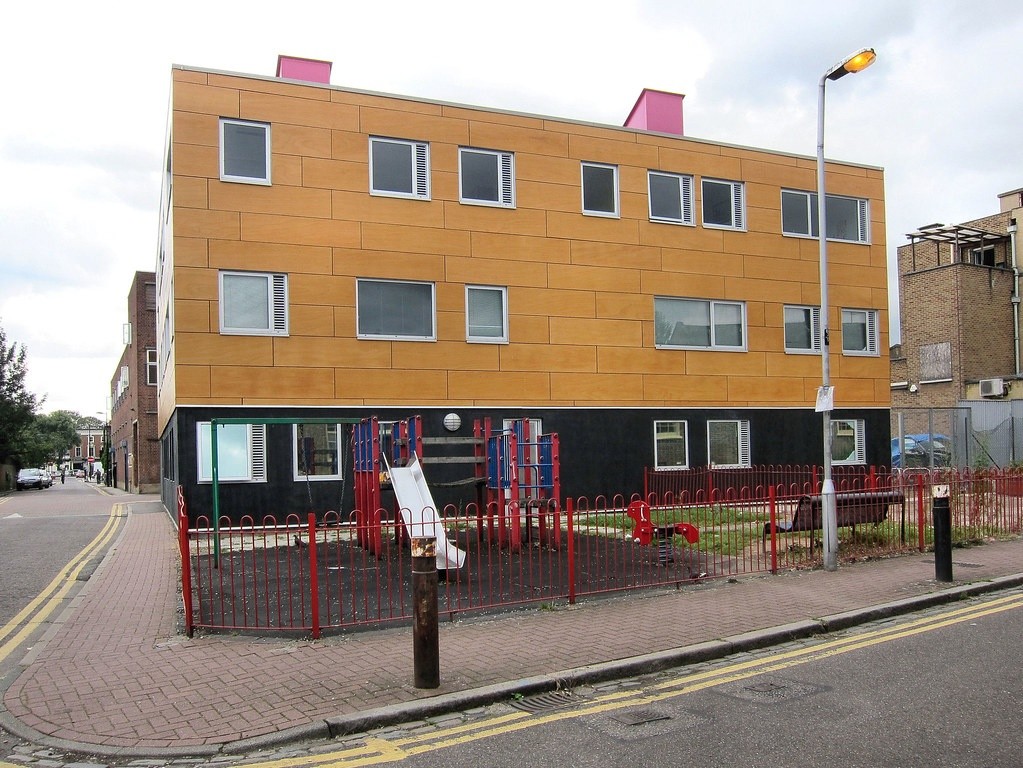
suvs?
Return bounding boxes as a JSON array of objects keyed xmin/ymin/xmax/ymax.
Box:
[{"xmin": 16, "ymin": 468, "xmax": 43, "ymax": 491}]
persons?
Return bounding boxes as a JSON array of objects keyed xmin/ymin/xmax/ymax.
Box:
[
  {"xmin": 103, "ymin": 471, "xmax": 107, "ymax": 485},
  {"xmin": 61, "ymin": 470, "xmax": 65, "ymax": 484},
  {"xmin": 94, "ymin": 469, "xmax": 101, "ymax": 485}
]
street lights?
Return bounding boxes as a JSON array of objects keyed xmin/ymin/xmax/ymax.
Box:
[
  {"xmin": 97, "ymin": 411, "xmax": 108, "ymax": 427},
  {"xmin": 817, "ymin": 46, "xmax": 876, "ymax": 575}
]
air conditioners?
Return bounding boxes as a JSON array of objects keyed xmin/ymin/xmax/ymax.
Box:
[{"xmin": 979, "ymin": 379, "xmax": 1005, "ymax": 399}]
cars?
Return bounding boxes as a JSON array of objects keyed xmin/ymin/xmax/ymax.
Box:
[
  {"xmin": 39, "ymin": 470, "xmax": 49, "ymax": 488},
  {"xmin": 54, "ymin": 469, "xmax": 86, "ymax": 478},
  {"xmin": 892, "ymin": 434, "xmax": 950, "ymax": 467},
  {"xmin": 47, "ymin": 472, "xmax": 52, "ymax": 486}
]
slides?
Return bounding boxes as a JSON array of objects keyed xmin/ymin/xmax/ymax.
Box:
[{"xmin": 378, "ymin": 450, "xmax": 468, "ymax": 571}]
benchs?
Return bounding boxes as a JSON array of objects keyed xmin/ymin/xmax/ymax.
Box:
[{"xmin": 762, "ymin": 489, "xmax": 906, "ymax": 549}]
